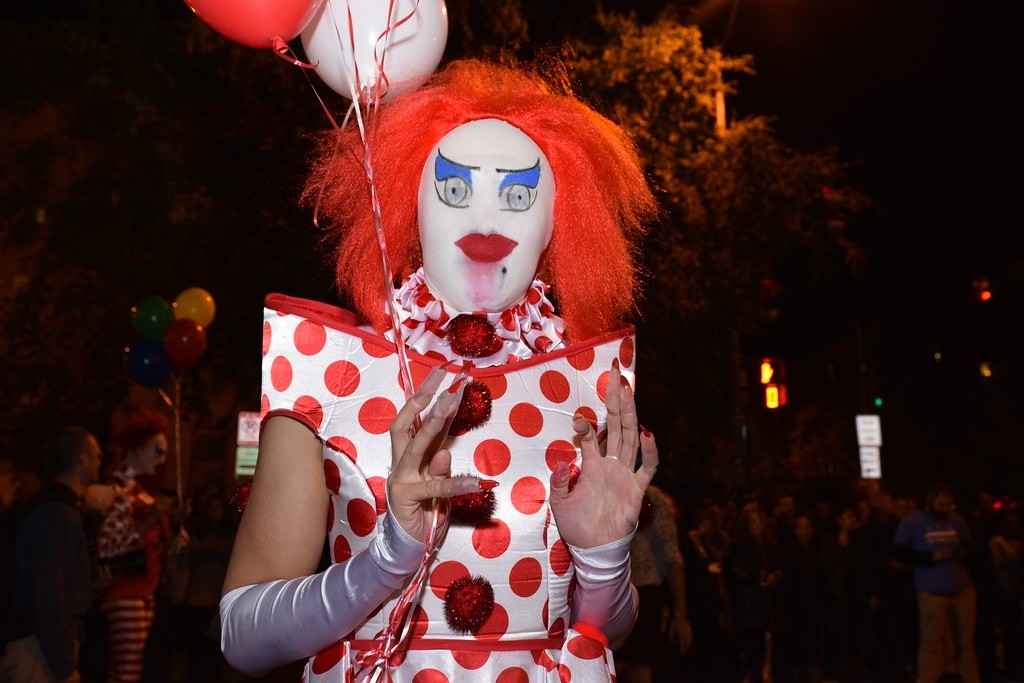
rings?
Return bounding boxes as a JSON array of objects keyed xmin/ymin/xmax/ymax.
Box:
[{"xmin": 606, "ymin": 455, "xmax": 619, "ymax": 459}]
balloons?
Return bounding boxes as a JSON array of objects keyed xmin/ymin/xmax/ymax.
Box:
[
  {"xmin": 173, "ymin": 288, "xmax": 216, "ymax": 326},
  {"xmin": 184, "ymin": 0, "xmax": 323, "ymax": 54},
  {"xmin": 127, "ymin": 342, "xmax": 170, "ymax": 387},
  {"xmin": 301, "ymin": 0, "xmax": 448, "ymax": 105},
  {"xmin": 164, "ymin": 320, "xmax": 208, "ymax": 370},
  {"xmin": 136, "ymin": 298, "xmax": 172, "ymax": 344}
]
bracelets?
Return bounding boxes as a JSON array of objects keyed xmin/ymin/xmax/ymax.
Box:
[{"xmin": 674, "ymin": 612, "xmax": 686, "ymax": 617}]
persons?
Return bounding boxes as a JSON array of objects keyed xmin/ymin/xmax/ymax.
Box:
[
  {"xmin": 220, "ymin": 65, "xmax": 660, "ymax": 683},
  {"xmin": 613, "ymin": 484, "xmax": 1024, "ymax": 683},
  {"xmin": 0, "ymin": 412, "xmax": 189, "ymax": 683}
]
